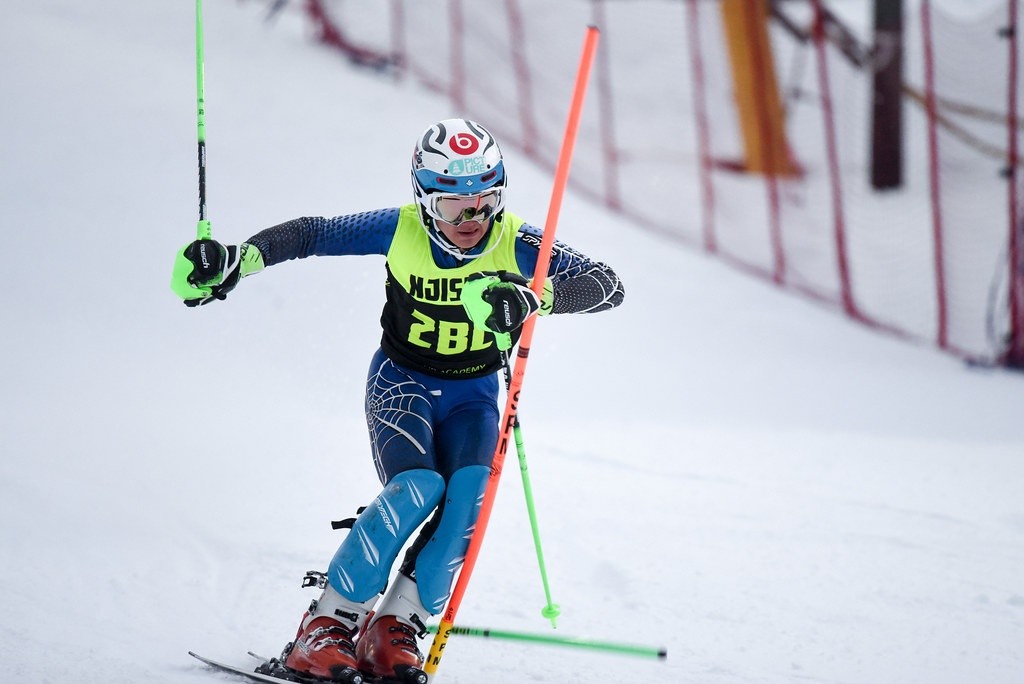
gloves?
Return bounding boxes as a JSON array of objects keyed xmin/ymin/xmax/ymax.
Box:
[
  {"xmin": 172, "ymin": 239, "xmax": 265, "ymax": 307},
  {"xmin": 460, "ymin": 270, "xmax": 554, "ymax": 334}
]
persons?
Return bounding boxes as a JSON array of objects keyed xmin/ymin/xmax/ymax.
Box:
[{"xmin": 172, "ymin": 119, "xmax": 624, "ymax": 684}]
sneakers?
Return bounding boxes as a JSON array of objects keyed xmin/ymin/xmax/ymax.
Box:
[
  {"xmin": 285, "ymin": 581, "xmax": 379, "ymax": 677},
  {"xmin": 355, "ymin": 570, "xmax": 431, "ymax": 676}
]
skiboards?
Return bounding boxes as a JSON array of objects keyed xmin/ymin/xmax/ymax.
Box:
[{"xmin": 187, "ymin": 622, "xmax": 664, "ymax": 684}]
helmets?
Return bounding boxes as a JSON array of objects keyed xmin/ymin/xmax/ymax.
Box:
[{"xmin": 411, "ymin": 119, "xmax": 507, "ymax": 226}]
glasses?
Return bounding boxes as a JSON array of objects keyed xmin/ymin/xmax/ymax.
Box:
[{"xmin": 417, "ymin": 186, "xmax": 507, "ymax": 227}]
